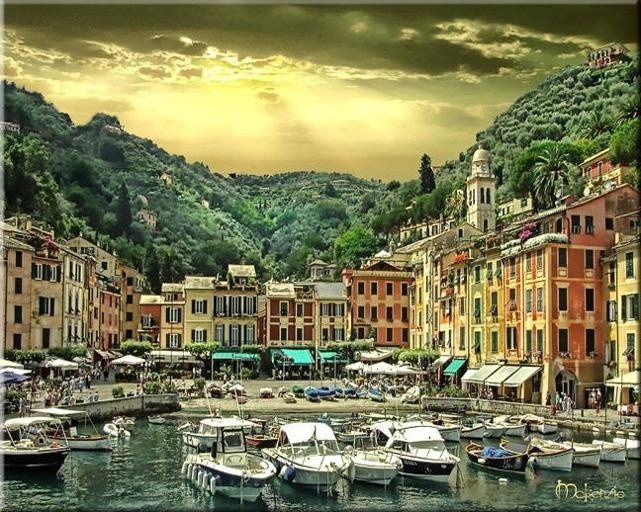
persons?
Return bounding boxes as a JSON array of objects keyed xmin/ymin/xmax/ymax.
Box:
[
  {"xmin": 545, "ymin": 388, "xmax": 602, "ymax": 415},
  {"xmin": 114, "ymin": 415, "xmax": 127, "ymax": 432},
  {"xmin": 354, "ymin": 374, "xmax": 451, "ymax": 396},
  {"xmin": 14, "ymin": 364, "xmax": 110, "ymax": 405}
]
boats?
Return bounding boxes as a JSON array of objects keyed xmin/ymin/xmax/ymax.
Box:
[
  {"xmin": 401, "ymin": 386, "xmax": 420, "ymax": 403},
  {"xmin": 278, "ymin": 385, "xmax": 384, "ymax": 402},
  {"xmin": 224, "ymin": 382, "xmax": 246, "ymax": 399},
  {"xmin": 177, "ymin": 413, "xmax": 462, "ymax": 502},
  {"xmin": 463, "ymin": 414, "xmax": 641, "ymax": 474},
  {"xmin": 0, "ymin": 408, "xmax": 112, "ymax": 479},
  {"xmin": 102, "ymin": 417, "xmax": 135, "ymax": 437},
  {"xmin": 207, "ymin": 381, "xmax": 223, "ymax": 397},
  {"xmin": 147, "ymin": 413, "xmax": 166, "ymax": 425},
  {"xmin": 259, "ymin": 387, "xmax": 273, "ymax": 398}
]
